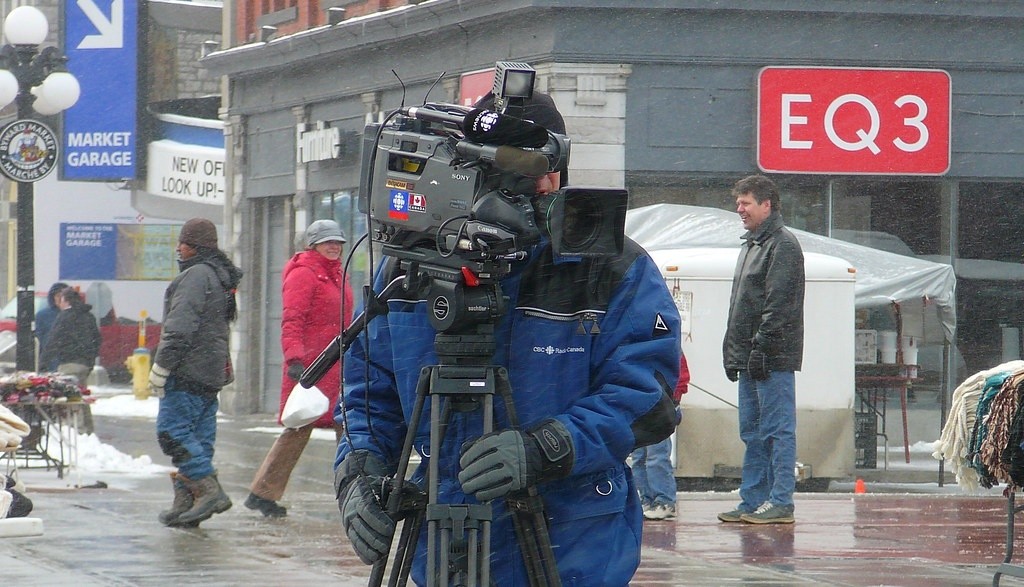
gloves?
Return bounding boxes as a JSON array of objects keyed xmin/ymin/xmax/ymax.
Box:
[
  {"xmin": 725, "ymin": 367, "xmax": 738, "ymax": 383},
  {"xmin": 458, "ymin": 419, "xmax": 576, "ymax": 502},
  {"xmin": 748, "ymin": 349, "xmax": 769, "ymax": 380},
  {"xmin": 288, "ymin": 363, "xmax": 304, "ymax": 381},
  {"xmin": 334, "ymin": 450, "xmax": 396, "ymax": 565},
  {"xmin": 146, "ymin": 363, "xmax": 171, "ymax": 398}
]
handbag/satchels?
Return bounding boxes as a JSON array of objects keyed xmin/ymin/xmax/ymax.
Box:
[{"xmin": 281, "ymin": 382, "xmax": 330, "ymax": 431}]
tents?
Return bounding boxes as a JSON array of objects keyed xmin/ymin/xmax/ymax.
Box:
[{"xmin": 625, "ymin": 203, "xmax": 957, "ymax": 486}]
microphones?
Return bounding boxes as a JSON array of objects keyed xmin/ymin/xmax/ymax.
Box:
[
  {"xmin": 456, "ymin": 141, "xmax": 549, "ymax": 177},
  {"xmin": 398, "ymin": 107, "xmax": 548, "ymax": 148}
]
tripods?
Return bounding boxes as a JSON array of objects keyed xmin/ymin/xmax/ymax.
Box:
[{"xmin": 300, "ymin": 244, "xmax": 563, "ymax": 587}]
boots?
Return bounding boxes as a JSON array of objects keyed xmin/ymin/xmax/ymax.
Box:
[
  {"xmin": 179, "ymin": 472, "xmax": 233, "ymax": 525},
  {"xmin": 158, "ymin": 473, "xmax": 199, "ymax": 527}
]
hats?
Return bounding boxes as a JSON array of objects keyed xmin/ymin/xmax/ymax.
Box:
[
  {"xmin": 307, "ymin": 220, "xmax": 346, "ymax": 244},
  {"xmin": 179, "ymin": 218, "xmax": 217, "ymax": 249},
  {"xmin": 471, "ymin": 86, "xmax": 569, "ymax": 186}
]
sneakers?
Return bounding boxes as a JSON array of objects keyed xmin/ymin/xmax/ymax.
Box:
[
  {"xmin": 718, "ymin": 508, "xmax": 743, "ymax": 522},
  {"xmin": 643, "ymin": 503, "xmax": 676, "ymax": 520},
  {"xmin": 245, "ymin": 492, "xmax": 287, "ymax": 516},
  {"xmin": 741, "ymin": 502, "xmax": 795, "ymax": 524}
]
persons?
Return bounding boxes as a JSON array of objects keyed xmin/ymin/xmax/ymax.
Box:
[
  {"xmin": 34, "ymin": 282, "xmax": 101, "ymax": 437},
  {"xmin": 718, "ymin": 173, "xmax": 805, "ymax": 522},
  {"xmin": 147, "ymin": 216, "xmax": 244, "ymax": 527},
  {"xmin": 631, "ymin": 351, "xmax": 691, "ymax": 519},
  {"xmin": 243, "ymin": 219, "xmax": 355, "ymax": 518},
  {"xmin": 333, "ymin": 82, "xmax": 682, "ymax": 586}
]
